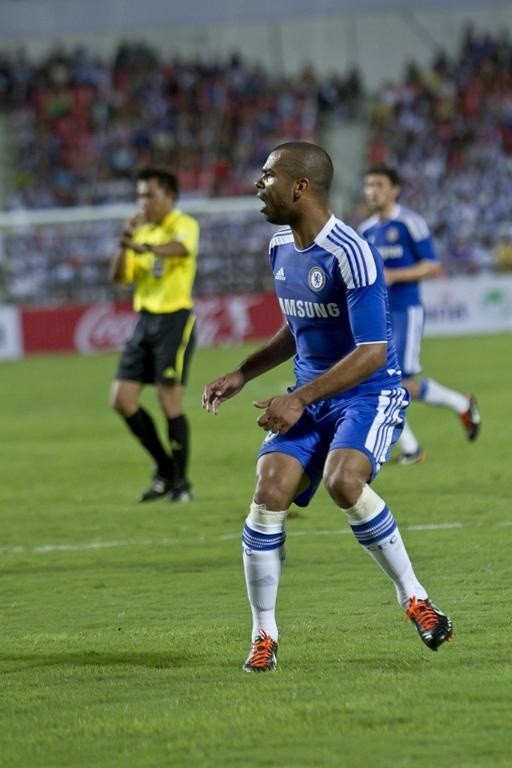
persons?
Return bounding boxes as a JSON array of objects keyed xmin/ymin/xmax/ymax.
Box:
[
  {"xmin": 107, "ymin": 168, "xmax": 194, "ymax": 504},
  {"xmin": 0, "ymin": 15, "xmax": 512, "ymax": 311},
  {"xmin": 356, "ymin": 157, "xmax": 481, "ymax": 465},
  {"xmin": 201, "ymin": 140, "xmax": 453, "ymax": 675}
]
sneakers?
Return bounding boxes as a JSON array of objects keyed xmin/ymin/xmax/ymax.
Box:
[
  {"xmin": 243, "ymin": 635, "xmax": 278, "ymax": 672},
  {"xmin": 390, "ymin": 449, "xmax": 426, "ymax": 465},
  {"xmin": 404, "ymin": 596, "xmax": 454, "ymax": 650},
  {"xmin": 459, "ymin": 391, "xmax": 481, "ymax": 441},
  {"xmin": 140, "ymin": 472, "xmax": 194, "ymax": 504}
]
